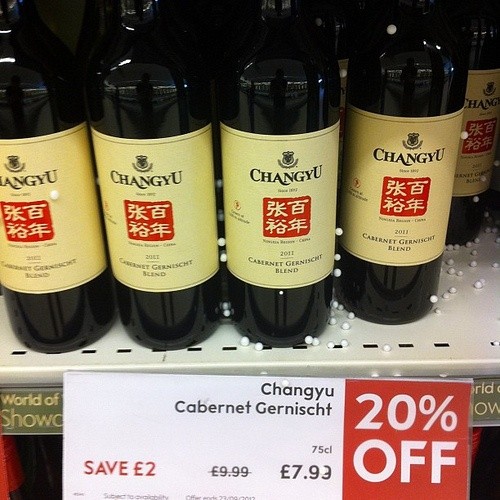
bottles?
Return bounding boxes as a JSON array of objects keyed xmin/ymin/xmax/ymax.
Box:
[
  {"xmin": 217, "ymin": 0, "xmax": 341, "ymax": 348},
  {"xmin": 81, "ymin": 1, "xmax": 224, "ymax": 350},
  {"xmin": 0, "ymin": 435, "xmax": 65, "ymax": 500},
  {"xmin": 0, "ymin": 1, "xmax": 117, "ymax": 353},
  {"xmin": 445, "ymin": 0, "xmax": 500, "ymax": 245},
  {"xmin": 338, "ymin": 0, "xmax": 469, "ymax": 325}
]
